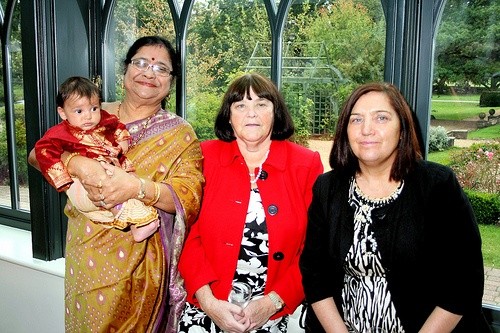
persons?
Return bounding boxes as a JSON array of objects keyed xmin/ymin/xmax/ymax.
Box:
[
  {"xmin": 297, "ymin": 82, "xmax": 485, "ymax": 333},
  {"xmin": 27, "ymin": 36, "xmax": 206, "ymax": 333},
  {"xmin": 178, "ymin": 73, "xmax": 324, "ymax": 333},
  {"xmin": 34, "ymin": 75, "xmax": 160, "ymax": 243}
]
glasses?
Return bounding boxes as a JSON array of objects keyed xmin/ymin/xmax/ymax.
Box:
[{"xmin": 129, "ymin": 59, "xmax": 175, "ymax": 78}]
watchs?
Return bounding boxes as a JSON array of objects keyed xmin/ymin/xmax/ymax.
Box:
[
  {"xmin": 134, "ymin": 177, "xmax": 147, "ymax": 200},
  {"xmin": 267, "ymin": 291, "xmax": 283, "ymax": 309}
]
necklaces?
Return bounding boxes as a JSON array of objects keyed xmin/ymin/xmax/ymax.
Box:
[
  {"xmin": 250, "ymin": 149, "xmax": 269, "ymax": 183},
  {"xmin": 353, "ymin": 173, "xmax": 401, "ymax": 203},
  {"xmin": 117, "ymin": 101, "xmax": 162, "ymax": 149}
]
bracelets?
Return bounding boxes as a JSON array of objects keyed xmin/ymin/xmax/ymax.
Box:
[
  {"xmin": 145, "ymin": 181, "xmax": 161, "ymax": 206},
  {"xmin": 64, "ymin": 152, "xmax": 81, "ymax": 178}
]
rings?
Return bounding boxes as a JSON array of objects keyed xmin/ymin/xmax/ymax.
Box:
[{"xmin": 98, "ymin": 179, "xmax": 106, "ymax": 207}]
